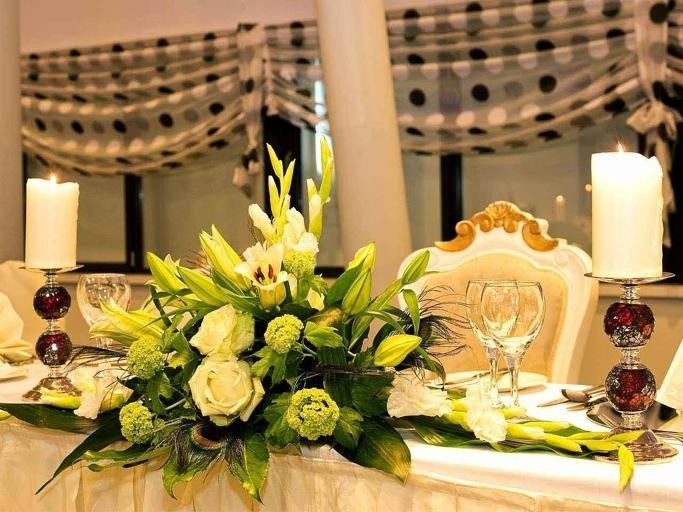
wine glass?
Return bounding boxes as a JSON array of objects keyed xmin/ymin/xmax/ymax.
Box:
[
  {"xmin": 464, "ymin": 281, "xmax": 546, "ymax": 407},
  {"xmin": 76, "ymin": 273, "xmax": 131, "ymax": 377}
]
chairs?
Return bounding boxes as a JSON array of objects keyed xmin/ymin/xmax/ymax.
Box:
[{"xmin": 398, "ymin": 201, "xmax": 599, "ymax": 384}]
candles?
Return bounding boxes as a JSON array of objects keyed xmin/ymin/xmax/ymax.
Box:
[
  {"xmin": 591, "ymin": 142, "xmax": 664, "ymax": 279},
  {"xmin": 24, "ymin": 175, "xmax": 80, "ymax": 269}
]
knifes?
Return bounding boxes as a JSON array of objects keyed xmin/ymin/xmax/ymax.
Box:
[
  {"xmin": 537, "ymin": 384, "xmax": 608, "ymax": 411},
  {"xmin": 436, "ymin": 368, "xmax": 509, "ymax": 386}
]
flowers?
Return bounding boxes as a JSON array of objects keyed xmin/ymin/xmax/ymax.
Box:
[{"xmin": 0, "ymin": 136, "xmax": 648, "ymax": 505}]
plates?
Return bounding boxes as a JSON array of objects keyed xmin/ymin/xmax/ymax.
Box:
[{"xmin": 427, "ymin": 369, "xmax": 547, "ymax": 392}]
